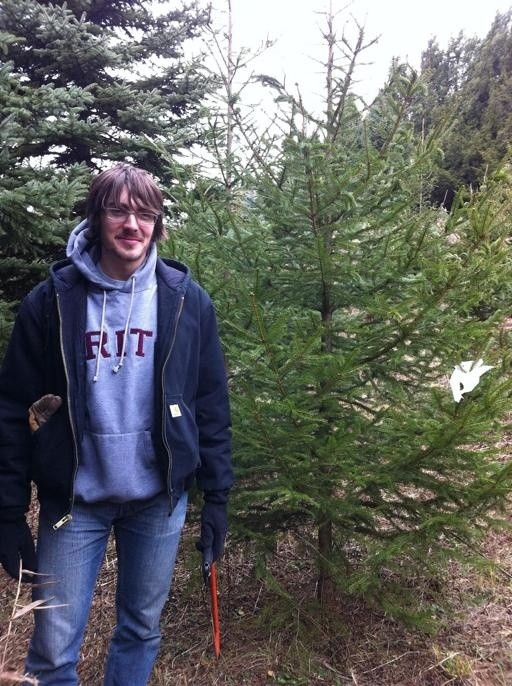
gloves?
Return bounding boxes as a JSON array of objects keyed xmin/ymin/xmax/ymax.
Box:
[
  {"xmin": 0, "ymin": 514, "xmax": 37, "ymax": 582},
  {"xmin": 196, "ymin": 502, "xmax": 227, "ymax": 562}
]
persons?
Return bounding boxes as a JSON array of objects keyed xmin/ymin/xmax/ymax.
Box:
[{"xmin": 0, "ymin": 159, "xmax": 233, "ymax": 685}]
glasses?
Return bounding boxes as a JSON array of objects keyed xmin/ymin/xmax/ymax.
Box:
[{"xmin": 102, "ymin": 207, "xmax": 159, "ymax": 226}]
